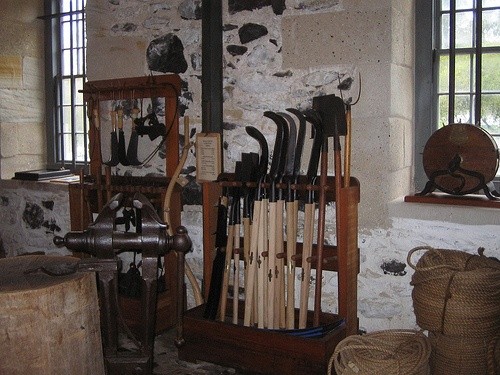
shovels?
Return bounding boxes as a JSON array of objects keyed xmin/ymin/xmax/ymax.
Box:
[
  {"xmin": 100, "ymin": 111, "xmax": 120, "ymax": 166},
  {"xmin": 116, "ymin": 107, "xmax": 130, "ymax": 166},
  {"xmin": 202, "ymin": 95, "xmax": 353, "ymax": 337},
  {"xmin": 127, "ymin": 107, "xmax": 145, "ymax": 167}
]
